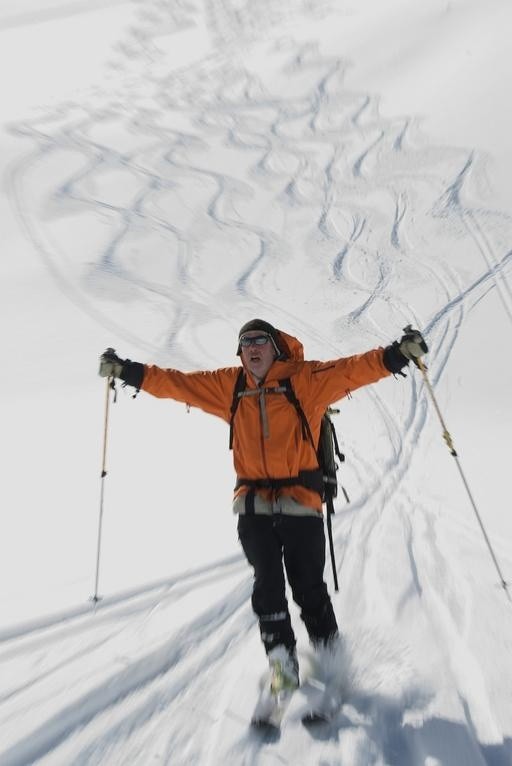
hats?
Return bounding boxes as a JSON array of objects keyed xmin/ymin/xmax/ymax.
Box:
[{"xmin": 236, "ymin": 319, "xmax": 282, "ymax": 357}]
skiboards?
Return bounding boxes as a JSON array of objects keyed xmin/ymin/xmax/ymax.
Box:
[{"xmin": 251, "ymin": 659, "xmax": 346, "ymax": 729}]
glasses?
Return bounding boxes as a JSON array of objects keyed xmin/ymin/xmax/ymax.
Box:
[{"xmin": 239, "ymin": 336, "xmax": 270, "ymax": 346}]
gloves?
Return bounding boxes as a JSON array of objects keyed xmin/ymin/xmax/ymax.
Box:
[
  {"xmin": 99, "ymin": 352, "xmax": 123, "ymax": 378},
  {"xmin": 393, "ymin": 330, "xmax": 429, "ymax": 361}
]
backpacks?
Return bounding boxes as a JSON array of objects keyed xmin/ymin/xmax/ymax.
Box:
[{"xmin": 229, "ymin": 364, "xmax": 338, "ymax": 502}]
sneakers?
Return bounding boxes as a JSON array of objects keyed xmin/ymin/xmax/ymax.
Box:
[{"xmin": 268, "ymin": 643, "xmax": 300, "ymax": 695}]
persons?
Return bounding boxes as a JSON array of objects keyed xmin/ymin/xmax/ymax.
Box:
[{"xmin": 96, "ymin": 318, "xmax": 431, "ymax": 731}]
